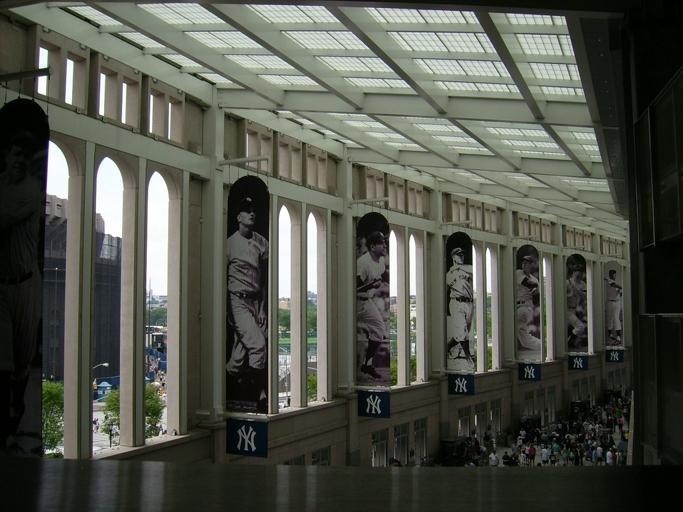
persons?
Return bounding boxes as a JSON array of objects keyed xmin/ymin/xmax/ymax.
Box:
[
  {"xmin": 222, "ymin": 191, "xmax": 268, "ymax": 406},
  {"xmin": 90, "ymin": 414, "xmax": 109, "ymax": 433},
  {"xmin": 391, "ymin": 385, "xmax": 629, "ymax": 465},
  {"xmin": 605, "ymin": 270, "xmax": 623, "ymax": 345},
  {"xmin": 514, "ymin": 255, "xmax": 540, "ymax": 352},
  {"xmin": 563, "ymin": 263, "xmax": 586, "ymax": 349},
  {"xmin": 444, "ymin": 247, "xmax": 475, "ymax": 361},
  {"xmin": 353, "ymin": 229, "xmax": 389, "ymax": 379}
]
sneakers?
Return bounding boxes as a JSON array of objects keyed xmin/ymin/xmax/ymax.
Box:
[
  {"xmin": 612, "ymin": 339, "xmax": 621, "ymax": 345},
  {"xmin": 464, "ymin": 355, "xmax": 475, "ymax": 367},
  {"xmin": 359, "ymin": 362, "xmax": 381, "ymax": 378},
  {"xmin": 447, "ymin": 351, "xmax": 456, "ymax": 359},
  {"xmin": 8, "ymin": 403, "xmax": 25, "ymax": 436}
]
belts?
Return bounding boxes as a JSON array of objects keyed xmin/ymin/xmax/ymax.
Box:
[
  {"xmin": 0, "ymin": 270, "xmax": 32, "ymax": 285},
  {"xmin": 456, "ymin": 297, "xmax": 473, "ymax": 302},
  {"xmin": 356, "ymin": 295, "xmax": 367, "ymax": 301},
  {"xmin": 516, "ymin": 301, "xmax": 524, "ymax": 304}
]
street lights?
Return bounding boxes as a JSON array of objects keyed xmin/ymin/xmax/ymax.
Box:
[
  {"xmin": 106, "ymin": 421, "xmax": 114, "ymax": 447},
  {"xmin": 90, "ymin": 362, "xmax": 109, "ymax": 371}
]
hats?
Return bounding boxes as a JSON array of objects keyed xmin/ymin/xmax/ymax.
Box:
[
  {"xmin": 8, "ymin": 129, "xmax": 39, "ymax": 150},
  {"xmin": 609, "ymin": 270, "xmax": 616, "ymax": 275},
  {"xmin": 233, "ymin": 194, "xmax": 257, "ymax": 213},
  {"xmin": 451, "ymin": 247, "xmax": 464, "ymax": 256},
  {"xmin": 521, "ymin": 256, "xmax": 532, "ymax": 263},
  {"xmin": 365, "ymin": 230, "xmax": 387, "ymax": 242},
  {"xmin": 573, "ymin": 262, "xmax": 584, "ymax": 272}
]
